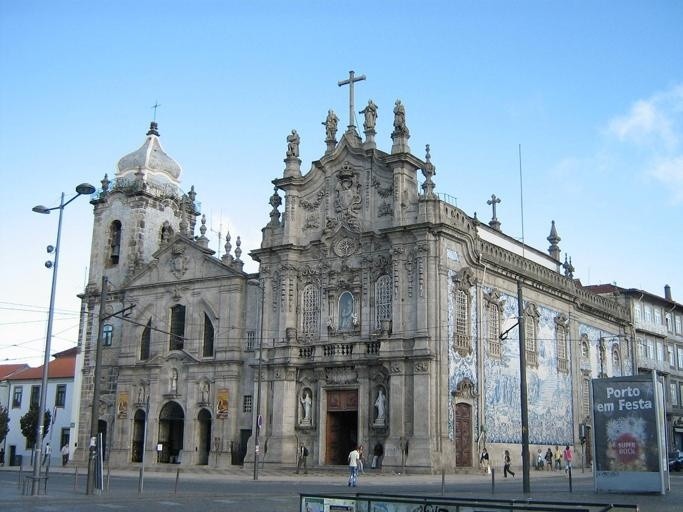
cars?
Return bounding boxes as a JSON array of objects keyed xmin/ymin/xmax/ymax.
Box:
[{"xmin": 670, "ymin": 452, "xmax": 683, "ymax": 473}]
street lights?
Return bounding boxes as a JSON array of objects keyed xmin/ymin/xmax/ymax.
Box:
[
  {"xmin": 248, "ymin": 279, "xmax": 264, "ymax": 480},
  {"xmin": 32, "ymin": 183, "xmax": 96, "ymax": 496},
  {"xmin": 87, "ymin": 304, "xmax": 137, "ymax": 495},
  {"xmin": 500, "ymin": 322, "xmax": 530, "ymax": 493}
]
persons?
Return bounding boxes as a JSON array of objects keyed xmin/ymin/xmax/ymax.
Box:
[
  {"xmin": 0, "ymin": 448, "xmax": 5, "ymax": 463},
  {"xmin": 375, "ymin": 390, "xmax": 385, "ymax": 418},
  {"xmin": 42, "ymin": 443, "xmax": 52, "ymax": 467},
  {"xmin": 301, "ymin": 394, "xmax": 311, "ymax": 416},
  {"xmin": 503, "ymin": 450, "xmax": 515, "ymax": 478},
  {"xmin": 537, "ymin": 445, "xmax": 573, "ymax": 474},
  {"xmin": 371, "ymin": 441, "xmax": 384, "ymax": 468},
  {"xmin": 479, "ymin": 448, "xmax": 489, "ymax": 472},
  {"xmin": 61, "ymin": 441, "xmax": 69, "ymax": 465},
  {"xmin": 286, "ymin": 99, "xmax": 409, "ymax": 159},
  {"xmin": 347, "ymin": 446, "xmax": 359, "ymax": 487},
  {"xmin": 358, "ymin": 445, "xmax": 366, "ymax": 475},
  {"xmin": 294, "ymin": 442, "xmax": 308, "ymax": 474}
]
